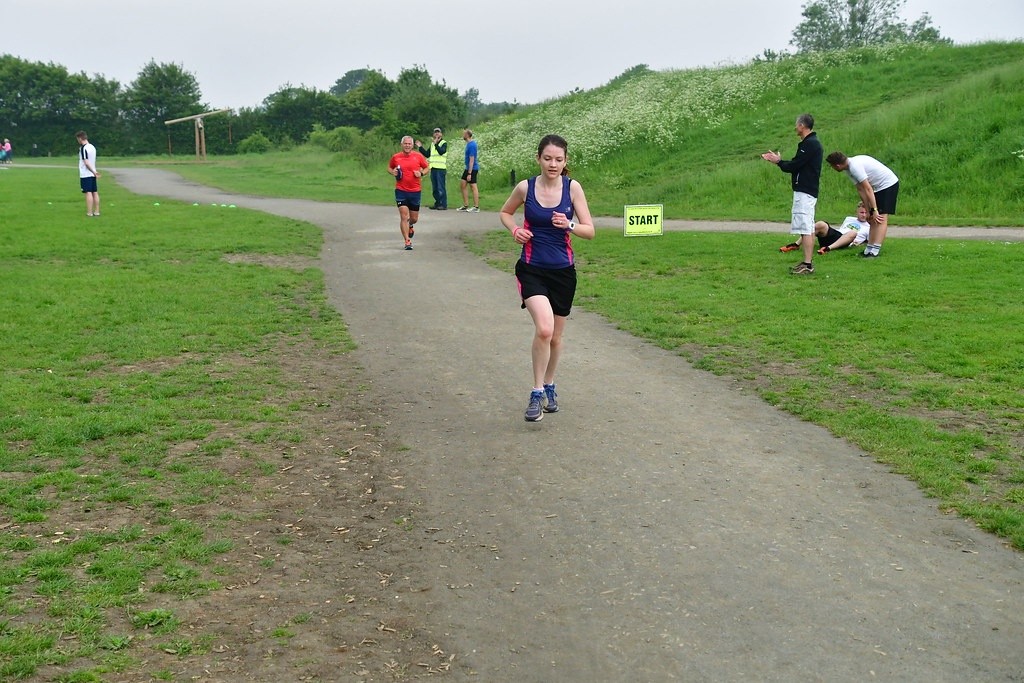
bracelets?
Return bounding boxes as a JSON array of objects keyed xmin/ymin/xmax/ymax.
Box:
[
  {"xmin": 870, "ymin": 208, "xmax": 878, "ymax": 211},
  {"xmin": 420, "ymin": 172, "xmax": 423, "ymax": 177},
  {"xmin": 468, "ymin": 172, "xmax": 471, "ymax": 175},
  {"xmin": 512, "ymin": 226, "xmax": 521, "ymax": 237}
]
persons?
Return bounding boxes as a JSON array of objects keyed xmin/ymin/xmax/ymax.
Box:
[
  {"xmin": 778, "ymin": 201, "xmax": 871, "ymax": 255},
  {"xmin": 75, "ymin": 131, "xmax": 100, "ymax": 216},
  {"xmin": 500, "ymin": 135, "xmax": 595, "ymax": 422},
  {"xmin": 0, "ymin": 143, "xmax": 8, "ymax": 164},
  {"xmin": 387, "ymin": 136, "xmax": 430, "ymax": 249},
  {"xmin": 761, "ymin": 114, "xmax": 823, "ymax": 275},
  {"xmin": 2, "ymin": 138, "xmax": 13, "ymax": 163},
  {"xmin": 456, "ymin": 130, "xmax": 480, "ymax": 213},
  {"xmin": 414, "ymin": 128, "xmax": 448, "ymax": 210},
  {"xmin": 826, "ymin": 151, "xmax": 899, "ymax": 258}
]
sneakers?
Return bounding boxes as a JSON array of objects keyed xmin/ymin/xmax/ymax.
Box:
[
  {"xmin": 456, "ymin": 205, "xmax": 468, "ymax": 211},
  {"xmin": 407, "ymin": 219, "xmax": 414, "ymax": 238},
  {"xmin": 404, "ymin": 240, "xmax": 414, "ymax": 250},
  {"xmin": 524, "ymin": 390, "xmax": 546, "ymax": 421},
  {"xmin": 466, "ymin": 205, "xmax": 480, "ymax": 212},
  {"xmin": 817, "ymin": 246, "xmax": 830, "ymax": 255},
  {"xmin": 789, "ymin": 263, "xmax": 814, "ymax": 274},
  {"xmin": 543, "ymin": 383, "xmax": 559, "ymax": 412},
  {"xmin": 779, "ymin": 242, "xmax": 800, "ymax": 253},
  {"xmin": 858, "ymin": 250, "xmax": 878, "ymax": 258}
]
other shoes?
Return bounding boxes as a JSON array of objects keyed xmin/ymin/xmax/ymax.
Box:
[{"xmin": 429, "ymin": 206, "xmax": 447, "ymax": 210}]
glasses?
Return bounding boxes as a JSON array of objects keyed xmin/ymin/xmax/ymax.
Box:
[{"xmin": 435, "ymin": 131, "xmax": 439, "ymax": 133}]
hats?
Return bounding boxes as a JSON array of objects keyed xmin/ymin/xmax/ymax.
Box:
[{"xmin": 434, "ymin": 128, "xmax": 441, "ymax": 132}]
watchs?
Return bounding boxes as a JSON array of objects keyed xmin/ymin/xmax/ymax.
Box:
[{"xmin": 565, "ymin": 221, "xmax": 575, "ymax": 232}]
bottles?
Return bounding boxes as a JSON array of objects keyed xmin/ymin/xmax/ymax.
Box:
[{"xmin": 396, "ymin": 165, "xmax": 402, "ymax": 180}]
